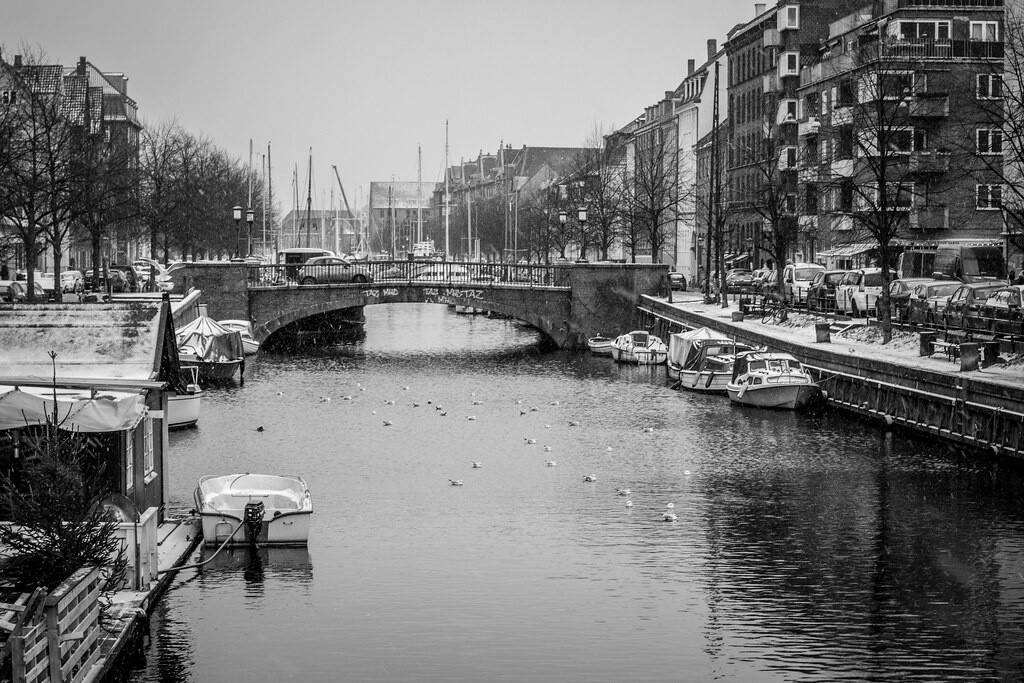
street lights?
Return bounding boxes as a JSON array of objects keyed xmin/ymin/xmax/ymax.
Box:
[
  {"xmin": 575, "ymin": 204, "xmax": 591, "ymax": 263},
  {"xmin": 230, "ymin": 205, "xmax": 245, "ymax": 262},
  {"xmin": 558, "ymin": 211, "xmax": 570, "ymax": 264},
  {"xmin": 245, "ymin": 208, "xmax": 255, "ymax": 259},
  {"xmin": 696, "ymin": 236, "xmax": 704, "ymax": 288}
]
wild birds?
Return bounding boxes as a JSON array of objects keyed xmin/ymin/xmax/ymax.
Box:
[{"xmin": 272, "ymin": 357, "xmax": 694, "ymax": 524}]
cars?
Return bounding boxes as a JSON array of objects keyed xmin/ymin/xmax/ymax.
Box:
[
  {"xmin": 666, "ymin": 271, "xmax": 687, "ymax": 293},
  {"xmin": 0, "ymin": 256, "xmax": 164, "ymax": 302},
  {"xmin": 702, "ymin": 254, "xmax": 1024, "ymax": 346},
  {"xmin": 295, "ymin": 256, "xmax": 375, "ymax": 285}
]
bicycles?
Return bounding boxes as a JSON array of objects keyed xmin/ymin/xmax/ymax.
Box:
[
  {"xmin": 761, "ymin": 300, "xmax": 789, "ymax": 325},
  {"xmin": 688, "ymin": 273, "xmax": 696, "ymax": 288}
]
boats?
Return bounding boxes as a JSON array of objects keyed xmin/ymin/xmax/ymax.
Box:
[
  {"xmin": 725, "ymin": 348, "xmax": 822, "ymax": 409},
  {"xmin": 587, "ymin": 333, "xmax": 615, "ymax": 356},
  {"xmin": 216, "ymin": 318, "xmax": 260, "ymax": 356},
  {"xmin": 192, "ymin": 472, "xmax": 313, "ymax": 548},
  {"xmin": 175, "ymin": 314, "xmax": 245, "ymax": 382},
  {"xmin": 610, "ymin": 330, "xmax": 668, "ymax": 364},
  {"xmin": 664, "ymin": 326, "xmax": 759, "ymax": 393},
  {"xmin": 168, "ymin": 365, "xmax": 203, "ymax": 430}
]
